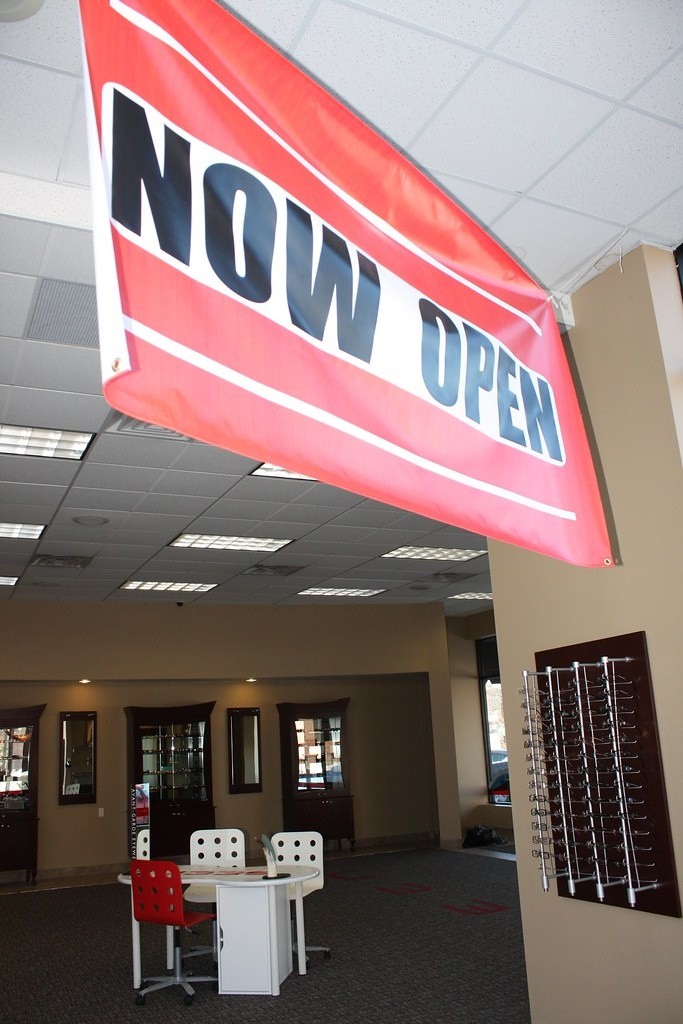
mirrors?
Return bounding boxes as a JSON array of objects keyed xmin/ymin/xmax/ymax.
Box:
[
  {"xmin": 226, "ymin": 708, "xmax": 263, "ymax": 794},
  {"xmin": 57, "ymin": 711, "xmax": 97, "ymax": 806}
]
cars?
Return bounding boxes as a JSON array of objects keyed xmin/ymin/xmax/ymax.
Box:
[{"xmin": 490, "ymin": 751, "xmax": 510, "ymax": 790}]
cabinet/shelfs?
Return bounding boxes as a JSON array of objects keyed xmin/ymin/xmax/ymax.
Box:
[
  {"xmin": 122, "ymin": 700, "xmax": 217, "ymax": 860},
  {"xmin": 276, "ymin": 697, "xmax": 356, "ymax": 854},
  {"xmin": 0, "ymin": 703, "xmax": 46, "ymax": 885},
  {"xmin": 215, "ymin": 885, "xmax": 294, "ymax": 994}
]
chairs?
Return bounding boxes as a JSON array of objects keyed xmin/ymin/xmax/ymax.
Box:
[{"xmin": 130, "ymin": 829, "xmax": 332, "ymax": 1005}]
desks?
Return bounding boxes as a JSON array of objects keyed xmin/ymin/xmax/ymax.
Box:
[{"xmin": 118, "ymin": 865, "xmax": 322, "ymax": 989}]
